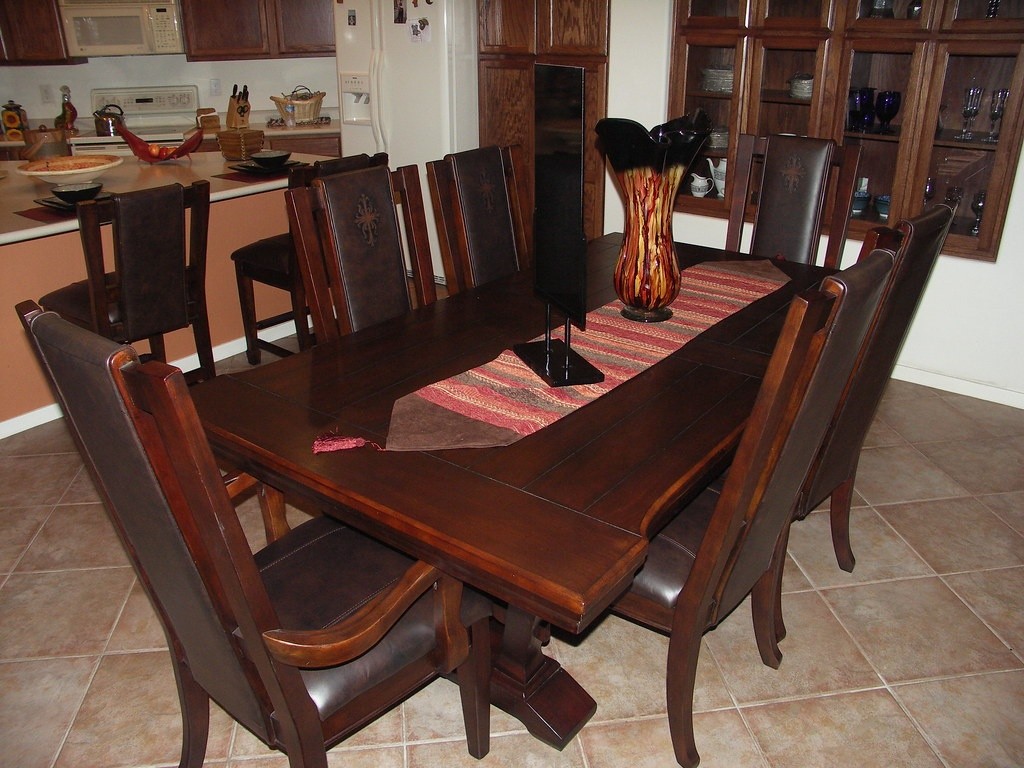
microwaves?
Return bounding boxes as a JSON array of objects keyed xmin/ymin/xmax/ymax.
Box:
[{"xmin": 57, "ymin": 0, "xmax": 185, "ymax": 58}]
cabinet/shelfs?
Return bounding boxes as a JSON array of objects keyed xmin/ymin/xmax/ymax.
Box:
[
  {"xmin": 269, "ymin": 136, "xmax": 342, "ymax": 160},
  {"xmin": 476, "ymin": 0, "xmax": 610, "ymax": 242},
  {"xmin": 181, "ymin": 0, "xmax": 337, "ymax": 63},
  {"xmin": 819, "ymin": 1, "xmax": 1024, "ymax": 262},
  {"xmin": 0, "ymin": 0, "xmax": 88, "ymax": 67},
  {"xmin": 662, "ymin": 1, "xmax": 827, "ymax": 230}
]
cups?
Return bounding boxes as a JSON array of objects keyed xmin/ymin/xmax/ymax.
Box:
[
  {"xmin": 945, "ymin": 185, "xmax": 963, "ymax": 210},
  {"xmin": 849, "ymin": 87, "xmax": 875, "ymax": 133}
]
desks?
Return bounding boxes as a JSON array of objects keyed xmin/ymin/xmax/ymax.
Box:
[{"xmin": 195, "ymin": 231, "xmax": 841, "ymax": 760}]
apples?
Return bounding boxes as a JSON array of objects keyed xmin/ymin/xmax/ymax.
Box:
[{"xmin": 148, "ymin": 144, "xmax": 177, "ymax": 158}]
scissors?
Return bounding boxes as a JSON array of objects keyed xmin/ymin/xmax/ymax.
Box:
[{"xmin": 237, "ymin": 105, "xmax": 250, "ymax": 117}]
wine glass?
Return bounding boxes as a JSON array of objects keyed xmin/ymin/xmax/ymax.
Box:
[
  {"xmin": 875, "ymin": 90, "xmax": 901, "ymax": 136},
  {"xmin": 981, "ymin": 89, "xmax": 1010, "ymax": 143},
  {"xmin": 923, "ymin": 176, "xmax": 936, "ymax": 208},
  {"xmin": 971, "ymin": 193, "xmax": 985, "ymax": 237},
  {"xmin": 953, "ymin": 87, "xmax": 984, "ymax": 140}
]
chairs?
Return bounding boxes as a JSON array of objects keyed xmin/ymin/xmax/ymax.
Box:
[{"xmin": 15, "ymin": 131, "xmax": 959, "ymax": 768}]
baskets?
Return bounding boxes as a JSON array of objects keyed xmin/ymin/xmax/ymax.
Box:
[{"xmin": 270, "ymin": 85, "xmax": 326, "ymax": 124}]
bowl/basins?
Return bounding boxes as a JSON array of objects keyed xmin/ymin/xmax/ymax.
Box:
[
  {"xmin": 786, "ymin": 74, "xmax": 813, "ymax": 100},
  {"xmin": 851, "ymin": 192, "xmax": 871, "ymax": 216},
  {"xmin": 700, "ymin": 67, "xmax": 733, "ymax": 94},
  {"xmin": 705, "ymin": 127, "xmax": 728, "ymax": 149},
  {"xmin": 250, "ymin": 150, "xmax": 292, "ymax": 168},
  {"xmin": 872, "ymin": 195, "xmax": 891, "ymax": 222},
  {"xmin": 215, "ymin": 128, "xmax": 265, "ymax": 161},
  {"xmin": 15, "ymin": 154, "xmax": 124, "ymax": 187},
  {"xmin": 50, "ymin": 183, "xmax": 103, "ymax": 205}
]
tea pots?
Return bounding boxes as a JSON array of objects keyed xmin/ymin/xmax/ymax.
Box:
[
  {"xmin": 93, "ymin": 103, "xmax": 125, "ymax": 137},
  {"xmin": 707, "ymin": 158, "xmax": 727, "ymax": 198}
]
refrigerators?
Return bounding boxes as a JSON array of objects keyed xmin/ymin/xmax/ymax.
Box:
[{"xmin": 333, "ymin": 0, "xmax": 478, "ymax": 286}]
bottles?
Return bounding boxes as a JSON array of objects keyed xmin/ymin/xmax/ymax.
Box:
[{"xmin": 1, "ymin": 100, "xmax": 30, "ymax": 142}]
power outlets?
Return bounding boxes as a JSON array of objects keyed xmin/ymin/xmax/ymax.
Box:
[
  {"xmin": 210, "ymin": 79, "xmax": 222, "ymax": 96},
  {"xmin": 40, "ymin": 84, "xmax": 55, "ymax": 103}
]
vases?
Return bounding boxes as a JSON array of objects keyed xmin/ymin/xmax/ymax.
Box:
[{"xmin": 595, "ymin": 112, "xmax": 708, "ymax": 324}]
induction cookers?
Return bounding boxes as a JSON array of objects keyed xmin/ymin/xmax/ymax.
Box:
[{"xmin": 66, "ymin": 85, "xmax": 200, "ymax": 144}]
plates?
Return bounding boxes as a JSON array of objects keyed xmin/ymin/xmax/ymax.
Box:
[
  {"xmin": 228, "ymin": 159, "xmax": 310, "ymax": 179},
  {"xmin": 33, "ymin": 191, "xmax": 117, "ymax": 215}
]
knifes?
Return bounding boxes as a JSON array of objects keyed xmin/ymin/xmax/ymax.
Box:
[{"xmin": 231, "ymin": 84, "xmax": 249, "ymax": 103}]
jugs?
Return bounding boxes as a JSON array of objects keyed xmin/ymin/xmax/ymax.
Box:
[{"xmin": 690, "ymin": 172, "xmax": 714, "ymax": 198}]
